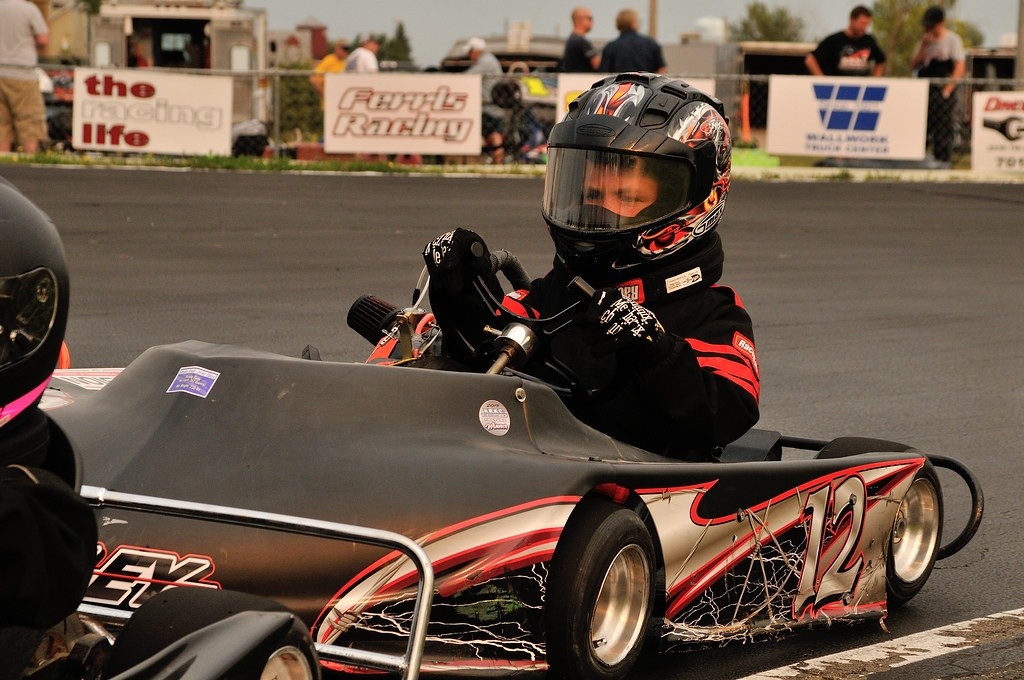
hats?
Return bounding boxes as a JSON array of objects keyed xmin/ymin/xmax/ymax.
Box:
[{"xmin": 465, "ymin": 37, "xmax": 486, "ymax": 50}]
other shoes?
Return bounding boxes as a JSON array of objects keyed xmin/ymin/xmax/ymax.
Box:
[{"xmin": 926, "ymin": 161, "xmax": 953, "ymax": 169}]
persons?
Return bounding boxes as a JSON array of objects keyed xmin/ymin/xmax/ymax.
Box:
[
  {"xmin": 911, "ymin": 6, "xmax": 967, "ymax": 169},
  {"xmin": 0, "ymin": 172, "xmax": 104, "ymax": 680},
  {"xmin": 805, "ymin": 5, "xmax": 887, "ymax": 77},
  {"xmin": 561, "ymin": 6, "xmax": 602, "ymax": 73},
  {"xmin": 462, "ymin": 38, "xmax": 507, "ymax": 164},
  {"xmin": 421, "ymin": 71, "xmax": 760, "ymax": 461},
  {"xmin": 0, "ymin": 0, "xmax": 50, "ymax": 156},
  {"xmin": 506, "ymin": 66, "xmax": 551, "ymax": 164},
  {"xmin": 600, "ymin": 8, "xmax": 670, "ymax": 78},
  {"xmin": 307, "ymin": 38, "xmax": 351, "ymax": 115},
  {"xmin": 343, "ymin": 31, "xmax": 385, "ymax": 76}
]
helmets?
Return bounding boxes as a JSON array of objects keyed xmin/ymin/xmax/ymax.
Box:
[
  {"xmin": 542, "ymin": 70, "xmax": 730, "ymax": 278},
  {"xmin": 0, "ymin": 178, "xmax": 72, "ymax": 433}
]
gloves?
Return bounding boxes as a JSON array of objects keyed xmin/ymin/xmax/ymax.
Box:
[
  {"xmin": 586, "ymin": 289, "xmax": 663, "ymax": 352},
  {"xmin": 424, "ymin": 228, "xmax": 494, "ymax": 298}
]
